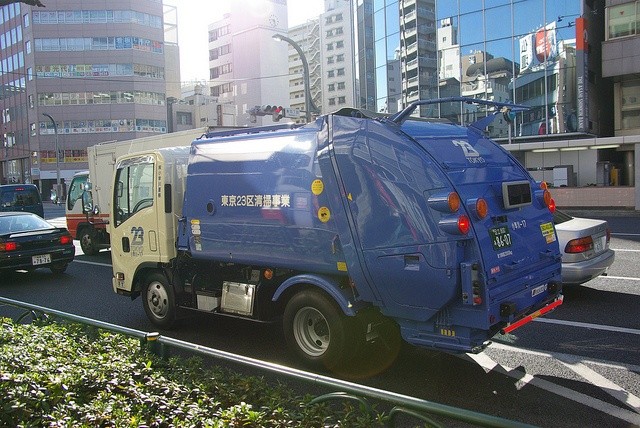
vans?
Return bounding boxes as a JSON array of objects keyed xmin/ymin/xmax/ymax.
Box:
[{"xmin": 0, "ymin": 185, "xmax": 44, "ymax": 219}]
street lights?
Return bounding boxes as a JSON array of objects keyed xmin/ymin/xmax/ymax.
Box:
[
  {"xmin": 272, "ymin": 34, "xmax": 322, "ymax": 123},
  {"xmin": 42, "ymin": 113, "xmax": 61, "ymax": 206}
]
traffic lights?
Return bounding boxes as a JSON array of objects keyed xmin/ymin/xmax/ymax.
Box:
[
  {"xmin": 272, "ymin": 114, "xmax": 283, "ymax": 122},
  {"xmin": 261, "ymin": 105, "xmax": 283, "ymax": 115}
]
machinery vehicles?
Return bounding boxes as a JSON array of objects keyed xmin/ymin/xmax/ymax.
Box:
[{"xmin": 109, "ymin": 98, "xmax": 564, "ymax": 373}]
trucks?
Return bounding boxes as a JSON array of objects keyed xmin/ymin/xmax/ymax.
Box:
[{"xmin": 65, "ymin": 126, "xmax": 253, "ymax": 255}]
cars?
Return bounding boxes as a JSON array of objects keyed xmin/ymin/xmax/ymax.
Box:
[
  {"xmin": 0, "ymin": 212, "xmax": 75, "ymax": 275},
  {"xmin": 552, "ymin": 208, "xmax": 615, "ymax": 286}
]
